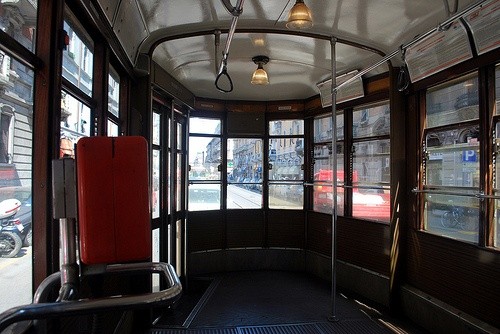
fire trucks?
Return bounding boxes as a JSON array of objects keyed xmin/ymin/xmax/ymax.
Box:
[{"xmin": 311, "ymin": 169, "xmax": 392, "ymax": 220}]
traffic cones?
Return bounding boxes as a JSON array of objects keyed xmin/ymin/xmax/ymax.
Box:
[{"xmin": 440, "ymin": 206, "xmax": 478, "ymax": 230}]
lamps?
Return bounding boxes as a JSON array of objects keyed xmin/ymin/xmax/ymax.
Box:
[
  {"xmin": 285, "ymin": 0, "xmax": 314, "ymax": 31},
  {"xmin": 248, "ymin": 55, "xmax": 270, "ymax": 86}
]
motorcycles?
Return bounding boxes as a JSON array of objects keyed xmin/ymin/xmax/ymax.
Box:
[{"xmin": 0, "ymin": 199, "xmax": 24, "ymax": 259}]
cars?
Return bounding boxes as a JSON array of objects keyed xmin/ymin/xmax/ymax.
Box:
[
  {"xmin": 226, "ymin": 172, "xmax": 304, "ymax": 202},
  {"xmin": 0, "ymin": 185, "xmax": 33, "ymax": 248}
]
